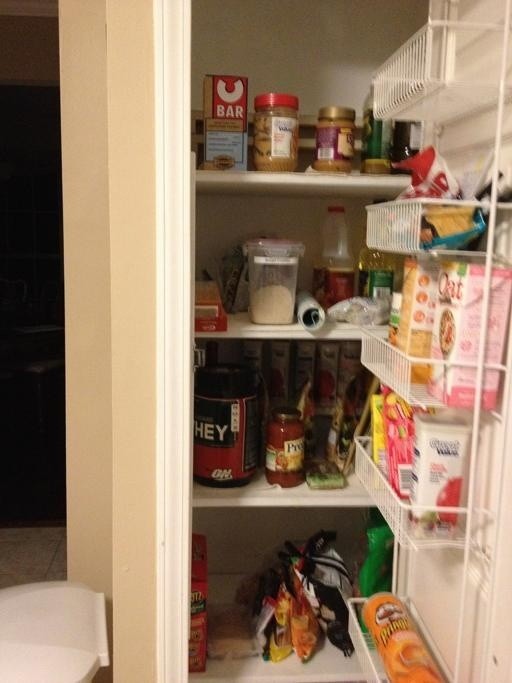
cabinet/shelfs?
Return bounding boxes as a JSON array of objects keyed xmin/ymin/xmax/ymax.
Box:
[
  {"xmin": 348, "ymin": 0, "xmax": 512, "ymax": 683},
  {"xmin": 188, "ymin": 151, "xmax": 413, "ymax": 683}
]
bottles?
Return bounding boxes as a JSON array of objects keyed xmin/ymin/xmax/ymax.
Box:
[
  {"xmin": 311, "ymin": 204, "xmax": 355, "ymax": 312},
  {"xmin": 361, "ymin": 76, "xmax": 421, "ymax": 176},
  {"xmin": 357, "ymin": 198, "xmax": 396, "ymax": 324}
]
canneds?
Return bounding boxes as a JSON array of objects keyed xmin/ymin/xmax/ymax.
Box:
[
  {"xmin": 252, "ymin": 92, "xmax": 300, "ymax": 171},
  {"xmin": 313, "ymin": 107, "xmax": 357, "ymax": 173},
  {"xmin": 265, "ymin": 407, "xmax": 306, "ymax": 488}
]
